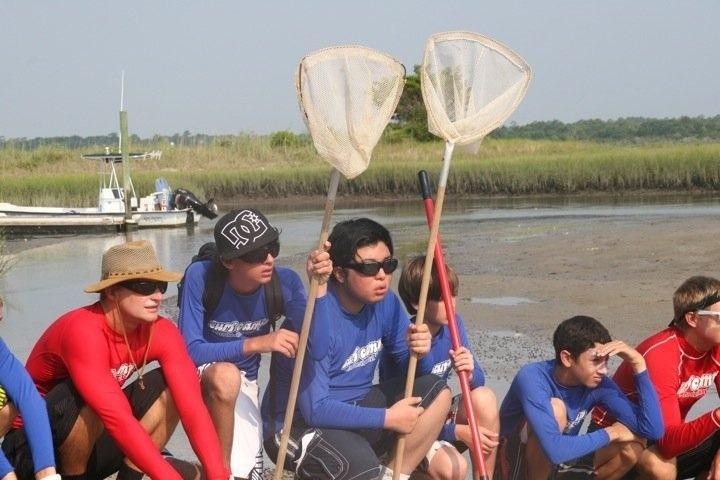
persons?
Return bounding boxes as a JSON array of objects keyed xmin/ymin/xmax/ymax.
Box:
[
  {"xmin": 0, "ymin": 300, "xmax": 61, "ymax": 480},
  {"xmin": 2, "ymin": 240, "xmax": 229, "ymax": 480},
  {"xmin": 260, "ymin": 217, "xmax": 453, "ymax": 480},
  {"xmin": 177, "ymin": 208, "xmax": 334, "ymax": 480},
  {"xmin": 588, "ymin": 275, "xmax": 720, "ymax": 480},
  {"xmin": 494, "ymin": 315, "xmax": 665, "ymax": 480},
  {"xmin": 378, "ymin": 255, "xmax": 501, "ymax": 480}
]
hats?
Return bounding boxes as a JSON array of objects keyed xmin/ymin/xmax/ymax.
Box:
[
  {"xmin": 84, "ymin": 239, "xmax": 183, "ymax": 293},
  {"xmin": 213, "ymin": 208, "xmax": 280, "ymax": 259}
]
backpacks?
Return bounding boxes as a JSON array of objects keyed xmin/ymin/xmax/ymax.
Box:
[{"xmin": 176, "ymin": 241, "xmax": 284, "ymax": 324}]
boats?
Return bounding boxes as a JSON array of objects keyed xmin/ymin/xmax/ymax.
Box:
[{"xmin": 0, "ymin": 70, "xmax": 205, "ymax": 226}]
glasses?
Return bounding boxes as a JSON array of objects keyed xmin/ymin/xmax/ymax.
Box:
[
  {"xmin": 120, "ymin": 280, "xmax": 169, "ymax": 295},
  {"xmin": 341, "ymin": 258, "xmax": 399, "ymax": 276},
  {"xmin": 240, "ymin": 241, "xmax": 280, "ymax": 263}
]
